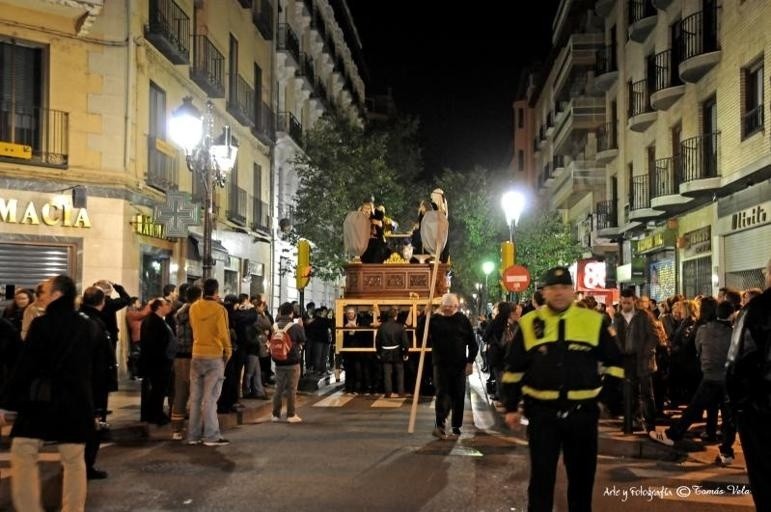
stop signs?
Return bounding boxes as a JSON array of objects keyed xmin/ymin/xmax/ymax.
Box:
[{"xmin": 502, "ymin": 266, "xmax": 530, "ymax": 292}]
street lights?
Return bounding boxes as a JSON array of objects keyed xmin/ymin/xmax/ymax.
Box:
[
  {"xmin": 166, "ymin": 94, "xmax": 240, "ymax": 282},
  {"xmin": 500, "ymin": 188, "xmax": 527, "ymax": 304},
  {"xmin": 472, "ymin": 261, "xmax": 495, "ymax": 321}
]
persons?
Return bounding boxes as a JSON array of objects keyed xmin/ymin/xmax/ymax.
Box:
[
  {"xmin": 505, "ymin": 266, "xmax": 627, "ymax": 511},
  {"xmin": 726, "ymin": 258, "xmax": 771, "ymax": 511},
  {"xmin": 358, "ymin": 188, "xmax": 451, "ymax": 263},
  {"xmin": 1, "ymin": 273, "xmax": 764, "ymax": 480},
  {"xmin": 651, "ymin": 266, "xmax": 659, "ymax": 285},
  {"xmin": 10, "ymin": 275, "xmax": 109, "ymax": 511}
]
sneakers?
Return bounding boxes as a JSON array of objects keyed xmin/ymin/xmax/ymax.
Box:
[
  {"xmin": 203, "ymin": 437, "xmax": 231, "ymax": 446},
  {"xmin": 718, "ymin": 450, "xmax": 732, "ymax": 466},
  {"xmin": 446, "ymin": 433, "xmax": 458, "ymax": 441},
  {"xmin": 287, "ymin": 414, "xmax": 302, "ymax": 423},
  {"xmin": 272, "ymin": 416, "xmax": 279, "ymax": 422},
  {"xmin": 188, "ymin": 438, "xmax": 202, "ymax": 445},
  {"xmin": 648, "ymin": 430, "xmax": 675, "ymax": 447},
  {"xmin": 432, "ymin": 427, "xmax": 446, "ymax": 440},
  {"xmin": 172, "ymin": 432, "xmax": 185, "ymax": 441}
]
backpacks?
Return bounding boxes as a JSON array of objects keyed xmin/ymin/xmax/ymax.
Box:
[{"xmin": 268, "ymin": 322, "xmax": 292, "ymax": 361}]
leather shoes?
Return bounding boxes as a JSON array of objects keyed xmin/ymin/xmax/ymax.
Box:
[{"xmin": 89, "ymin": 470, "xmax": 107, "ymax": 479}]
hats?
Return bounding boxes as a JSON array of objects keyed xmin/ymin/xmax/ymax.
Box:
[{"xmin": 540, "ymin": 266, "xmax": 572, "ymax": 287}]
